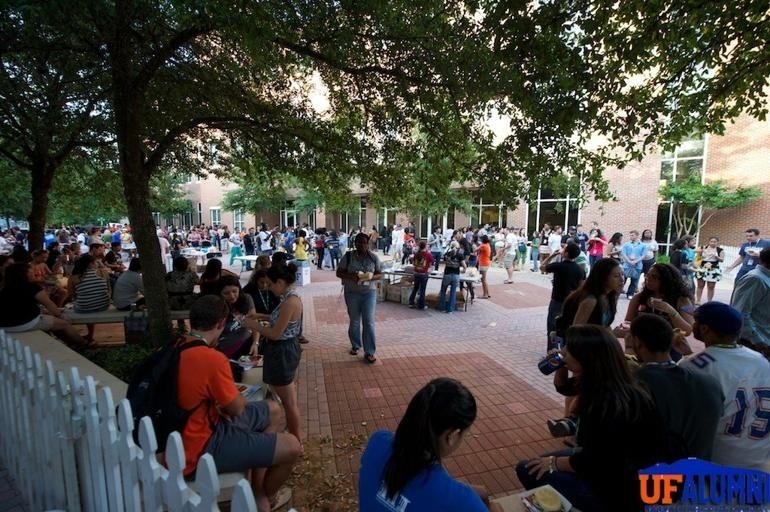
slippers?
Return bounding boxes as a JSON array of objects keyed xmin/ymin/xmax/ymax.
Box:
[
  {"xmin": 365, "ymin": 354, "xmax": 376, "ymax": 362},
  {"xmin": 351, "ymin": 349, "xmax": 359, "ymax": 355}
]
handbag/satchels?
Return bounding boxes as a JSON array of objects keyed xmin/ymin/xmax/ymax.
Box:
[{"xmin": 519, "ymin": 242, "xmax": 527, "ymax": 252}]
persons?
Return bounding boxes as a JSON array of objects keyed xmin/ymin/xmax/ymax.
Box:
[{"xmin": 0, "ymin": 220, "xmax": 770, "ymax": 511}]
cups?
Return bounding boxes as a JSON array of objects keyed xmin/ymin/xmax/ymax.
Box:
[{"xmin": 622, "ymin": 321, "xmax": 632, "ymax": 329}]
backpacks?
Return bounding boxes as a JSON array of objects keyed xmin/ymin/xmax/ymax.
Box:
[{"xmin": 116, "ymin": 338, "xmax": 209, "ymax": 455}]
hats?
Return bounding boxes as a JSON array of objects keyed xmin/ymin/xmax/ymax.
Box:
[
  {"xmin": 681, "ymin": 301, "xmax": 741, "ymax": 332},
  {"xmin": 281, "ymin": 251, "xmax": 295, "ymax": 260},
  {"xmin": 90, "ymin": 238, "xmax": 106, "ymax": 245}
]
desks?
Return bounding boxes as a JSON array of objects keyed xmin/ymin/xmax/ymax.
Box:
[
  {"xmin": 120, "ymin": 242, "xmax": 287, "ymax": 279},
  {"xmin": 338, "ymin": 265, "xmax": 483, "ymax": 311}
]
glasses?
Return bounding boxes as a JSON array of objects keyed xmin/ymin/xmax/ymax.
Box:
[
  {"xmin": 94, "ymin": 246, "xmax": 104, "ymax": 249},
  {"xmin": 643, "ymin": 272, "xmax": 657, "ymax": 280}
]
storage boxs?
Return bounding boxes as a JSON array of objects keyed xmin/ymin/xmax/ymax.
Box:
[
  {"xmin": 371, "ymin": 278, "xmax": 421, "ymax": 306},
  {"xmin": 289, "ymin": 259, "xmax": 311, "ymax": 287}
]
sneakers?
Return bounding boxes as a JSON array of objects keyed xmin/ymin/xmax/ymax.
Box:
[
  {"xmin": 270, "ymin": 488, "xmax": 293, "ymax": 512},
  {"xmin": 545, "ymin": 416, "xmax": 577, "ymax": 439}
]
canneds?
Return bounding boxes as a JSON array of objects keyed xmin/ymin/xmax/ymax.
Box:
[{"xmin": 538, "ymin": 350, "xmax": 563, "ymax": 376}]
